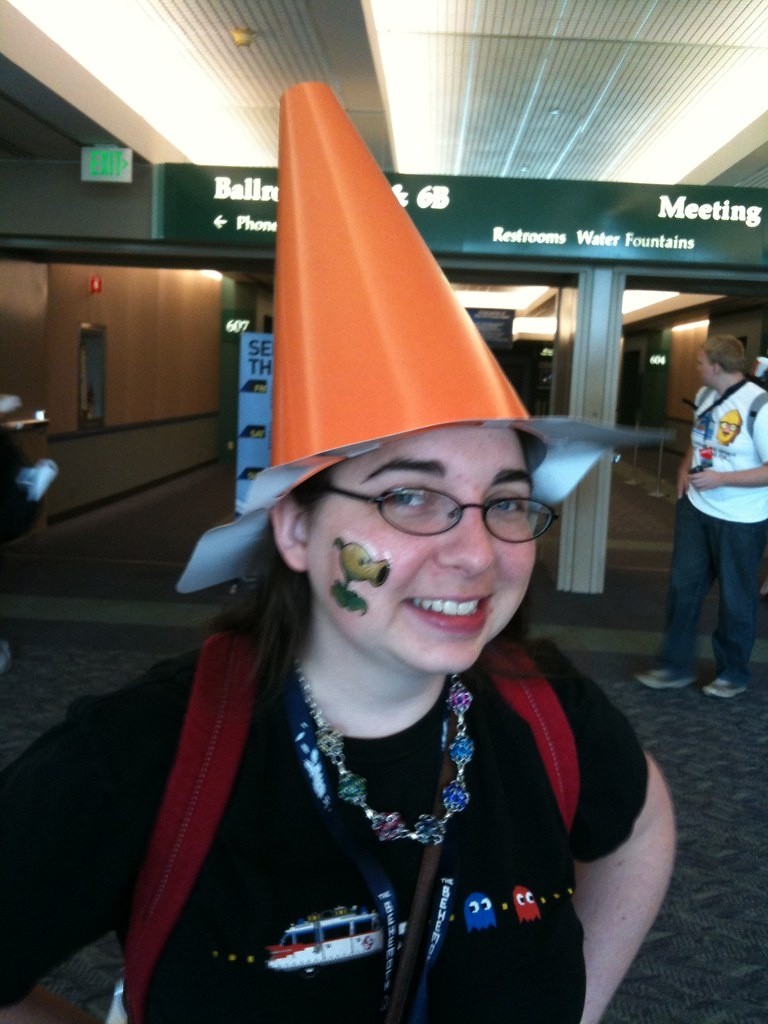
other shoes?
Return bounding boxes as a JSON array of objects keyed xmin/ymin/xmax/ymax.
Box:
[
  {"xmin": 702, "ymin": 678, "xmax": 749, "ymax": 698},
  {"xmin": 635, "ymin": 666, "xmax": 698, "ymax": 691}
]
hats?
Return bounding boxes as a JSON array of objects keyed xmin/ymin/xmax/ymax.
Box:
[{"xmin": 176, "ymin": 78, "xmax": 678, "ymax": 600}]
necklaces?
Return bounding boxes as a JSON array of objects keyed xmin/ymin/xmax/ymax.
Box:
[{"xmin": 292, "ymin": 651, "xmax": 473, "ymax": 846}]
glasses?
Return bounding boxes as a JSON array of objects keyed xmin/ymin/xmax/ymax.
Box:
[{"xmin": 320, "ymin": 483, "xmax": 560, "ymax": 544}]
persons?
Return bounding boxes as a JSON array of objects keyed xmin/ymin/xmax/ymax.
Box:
[
  {"xmin": 638, "ymin": 335, "xmax": 768, "ymax": 697},
  {"xmin": 1, "ymin": 352, "xmax": 676, "ymax": 1023}
]
